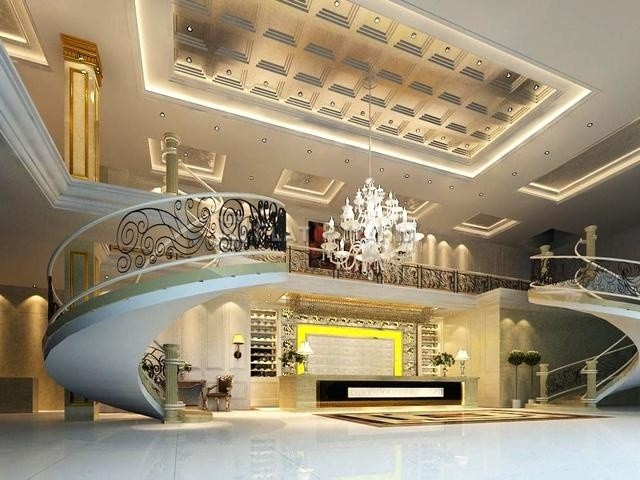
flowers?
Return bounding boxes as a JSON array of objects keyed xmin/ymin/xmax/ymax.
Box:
[{"xmin": 433, "ymin": 352, "xmax": 455, "ymax": 369}]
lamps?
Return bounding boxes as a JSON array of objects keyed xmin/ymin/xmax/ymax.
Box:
[
  {"xmin": 454, "ymin": 349, "xmax": 470, "ymax": 377},
  {"xmin": 296, "ymin": 342, "xmax": 314, "ymax": 373},
  {"xmin": 319, "ymin": 61, "xmax": 425, "ymax": 277},
  {"xmin": 232, "ymin": 335, "xmax": 244, "ymax": 359}
]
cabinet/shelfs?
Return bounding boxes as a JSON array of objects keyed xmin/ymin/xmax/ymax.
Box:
[{"xmin": 250, "ymin": 309, "xmax": 278, "ymax": 407}]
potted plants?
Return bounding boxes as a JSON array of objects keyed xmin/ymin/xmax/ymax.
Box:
[{"xmin": 507, "ymin": 350, "xmax": 542, "ymax": 409}]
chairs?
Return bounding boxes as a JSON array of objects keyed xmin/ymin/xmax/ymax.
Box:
[{"xmin": 203, "ymin": 375, "xmax": 234, "ymax": 411}]
